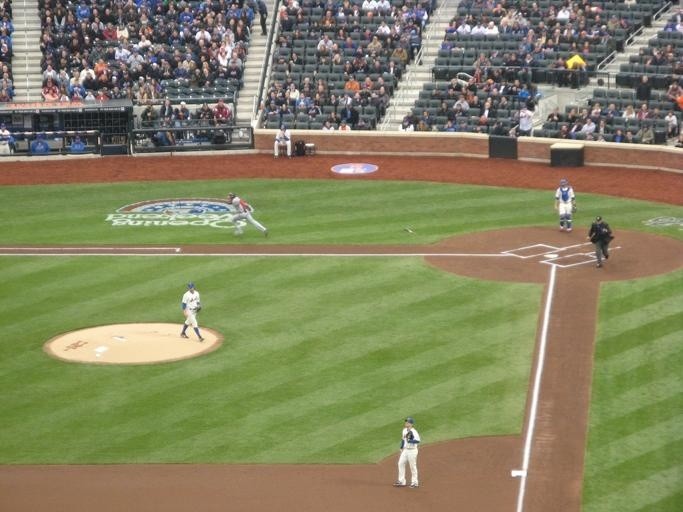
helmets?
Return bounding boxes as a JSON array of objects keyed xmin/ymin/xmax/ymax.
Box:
[{"xmin": 226, "ymin": 192, "xmax": 235, "ymax": 204}]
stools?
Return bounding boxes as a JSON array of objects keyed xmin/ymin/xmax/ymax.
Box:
[{"xmin": 304, "ymin": 144, "xmax": 316, "ymax": 157}]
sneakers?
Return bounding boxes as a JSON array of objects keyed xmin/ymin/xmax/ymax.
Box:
[
  {"xmin": 409, "ymin": 485, "xmax": 415, "ymax": 488},
  {"xmin": 274, "ymin": 156, "xmax": 279, "ymax": 159},
  {"xmin": 181, "ymin": 333, "xmax": 188, "ymax": 338},
  {"xmin": 560, "ymin": 226, "xmax": 564, "ymax": 231},
  {"xmin": 566, "ymin": 228, "xmax": 572, "ymax": 233},
  {"xmin": 595, "ymin": 264, "xmax": 602, "ymax": 267},
  {"xmin": 393, "ymin": 482, "xmax": 402, "ymax": 485},
  {"xmin": 264, "ymin": 229, "xmax": 269, "ymax": 237},
  {"xmin": 606, "ymin": 255, "xmax": 609, "ymax": 259},
  {"xmin": 200, "ymin": 337, "xmax": 204, "ymax": 341},
  {"xmin": 288, "ymin": 155, "xmax": 291, "ymax": 159}
]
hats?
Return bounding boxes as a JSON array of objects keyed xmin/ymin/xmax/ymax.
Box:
[
  {"xmin": 280, "ymin": 125, "xmax": 287, "ymax": 130},
  {"xmin": 560, "ymin": 178, "xmax": 567, "ymax": 185},
  {"xmin": 188, "ymin": 281, "xmax": 194, "ymax": 289},
  {"xmin": 405, "ymin": 417, "xmax": 414, "ymax": 424},
  {"xmin": 596, "ymin": 216, "xmax": 601, "ymax": 221}
]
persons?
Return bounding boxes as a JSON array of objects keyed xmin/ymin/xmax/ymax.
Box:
[
  {"xmin": 584, "ymin": 214, "xmax": 611, "ymax": 270},
  {"xmin": 392, "ymin": 417, "xmax": 421, "ymax": 489},
  {"xmin": 227, "ymin": 191, "xmax": 268, "ymax": 238},
  {"xmin": 553, "ymin": 178, "xmax": 577, "ymax": 233},
  {"xmin": 398, "ymin": 1, "xmax": 683, "ymax": 148},
  {"xmin": 0, "ymin": 1, "xmax": 256, "ymax": 155},
  {"xmin": 256, "ymin": 1, "xmax": 436, "ymax": 157},
  {"xmin": 179, "ymin": 282, "xmax": 204, "ymax": 343}
]
